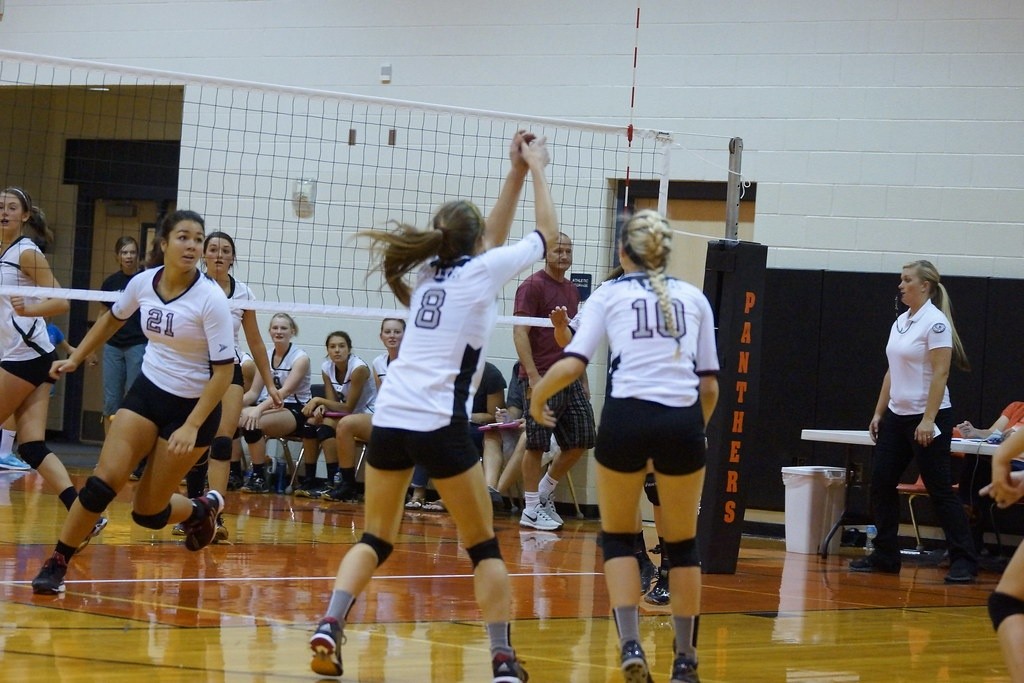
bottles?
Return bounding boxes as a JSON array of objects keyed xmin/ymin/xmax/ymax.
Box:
[
  {"xmin": 866, "ymin": 524, "xmax": 879, "ymax": 556},
  {"xmin": 245, "ymin": 463, "xmax": 253, "ymax": 486},
  {"xmin": 276, "ymin": 462, "xmax": 287, "ymax": 493},
  {"xmin": 333, "ymin": 468, "xmax": 343, "ymax": 491}
]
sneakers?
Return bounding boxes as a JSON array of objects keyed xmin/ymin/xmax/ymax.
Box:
[
  {"xmin": 640, "ymin": 563, "xmax": 659, "ymax": 596},
  {"xmin": 180, "ymin": 489, "xmax": 224, "ymax": 552},
  {"xmin": 643, "ymin": 566, "xmax": 671, "ymax": 605},
  {"xmin": 305, "ymin": 482, "xmax": 337, "ymax": 498},
  {"xmin": 518, "ymin": 530, "xmax": 561, "ymax": 552},
  {"xmin": 519, "ymin": 504, "xmax": 562, "ymax": 531},
  {"xmin": 32, "ymin": 556, "xmax": 67, "ymax": 593},
  {"xmin": 0, "ymin": 454, "xmax": 31, "ymax": 470},
  {"xmin": 308, "ymin": 616, "xmax": 346, "ymax": 676},
  {"xmin": 539, "ymin": 491, "xmax": 565, "ymax": 525},
  {"xmin": 670, "ymin": 653, "xmax": 701, "ymax": 683},
  {"xmin": 296, "ymin": 481, "xmax": 316, "ymax": 496},
  {"xmin": 621, "ymin": 640, "xmax": 654, "ymax": 683},
  {"xmin": 491, "ymin": 651, "xmax": 529, "ymax": 683},
  {"xmin": 74, "ymin": 515, "xmax": 108, "ymax": 554},
  {"xmin": 226, "ymin": 469, "xmax": 244, "ymax": 491},
  {"xmin": 240, "ymin": 471, "xmax": 269, "ymax": 493},
  {"xmin": 322, "ymin": 480, "xmax": 359, "ymax": 503}
]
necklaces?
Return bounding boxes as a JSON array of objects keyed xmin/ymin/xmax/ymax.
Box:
[{"xmin": 894, "ymin": 292, "xmax": 913, "ymax": 334}]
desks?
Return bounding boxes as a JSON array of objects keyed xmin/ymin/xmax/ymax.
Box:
[{"xmin": 801, "ymin": 428, "xmax": 1024, "ymax": 559}]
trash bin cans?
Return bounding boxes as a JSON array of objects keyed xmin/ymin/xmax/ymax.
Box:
[{"xmin": 781, "ymin": 466, "xmax": 846, "ymax": 554}]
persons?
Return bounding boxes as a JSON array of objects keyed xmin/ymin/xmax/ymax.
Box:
[
  {"xmin": 148, "ymin": 231, "xmax": 284, "ymax": 540},
  {"xmin": 404, "ymin": 363, "xmax": 507, "ymax": 512},
  {"xmin": 31, "ymin": 209, "xmax": 237, "ymax": 593},
  {"xmin": 848, "ymin": 259, "xmax": 978, "ymax": 584},
  {"xmin": 512, "ymin": 231, "xmax": 598, "ymax": 531},
  {"xmin": 294, "ymin": 329, "xmax": 379, "ymax": 501},
  {"xmin": 309, "ymin": 130, "xmax": 559, "ymax": 683},
  {"xmin": 226, "ymin": 314, "xmax": 314, "ymax": 494},
  {"xmin": 324, "ymin": 318, "xmax": 408, "ymax": 502},
  {"xmin": 0, "ymin": 188, "xmax": 109, "ymax": 555},
  {"xmin": 85, "ymin": 236, "xmax": 151, "ymax": 481},
  {"xmin": 987, "ymin": 425, "xmax": 1024, "ymax": 683},
  {"xmin": 529, "ymin": 209, "xmax": 721, "ymax": 683},
  {"xmin": 497, "ymin": 361, "xmax": 593, "ymax": 517},
  {"xmin": 956, "ymin": 401, "xmax": 1024, "ymax": 550}
]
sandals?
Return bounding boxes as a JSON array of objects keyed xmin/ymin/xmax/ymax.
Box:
[
  {"xmin": 404, "ymin": 497, "xmax": 424, "ymax": 509},
  {"xmin": 424, "ymin": 499, "xmax": 446, "ymax": 512}
]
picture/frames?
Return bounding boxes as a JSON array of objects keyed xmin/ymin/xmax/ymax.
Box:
[{"xmin": 138, "ymin": 222, "xmax": 156, "ymax": 271}]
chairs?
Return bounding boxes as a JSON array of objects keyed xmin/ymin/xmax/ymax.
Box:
[
  {"xmin": 230, "ymin": 354, "xmax": 586, "ymax": 520},
  {"xmin": 896, "ymin": 427, "xmax": 1024, "ymax": 552}
]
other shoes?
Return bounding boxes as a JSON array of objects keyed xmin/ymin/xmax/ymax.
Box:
[
  {"xmin": 171, "ymin": 523, "xmax": 185, "ymax": 534},
  {"xmin": 944, "ymin": 568, "xmax": 979, "ymax": 583},
  {"xmin": 181, "ymin": 478, "xmax": 188, "ymax": 485},
  {"xmin": 851, "ymin": 557, "xmax": 897, "ymax": 572},
  {"xmin": 212, "ymin": 510, "xmax": 229, "ymax": 544},
  {"xmin": 130, "ymin": 472, "xmax": 142, "ymax": 482}
]
